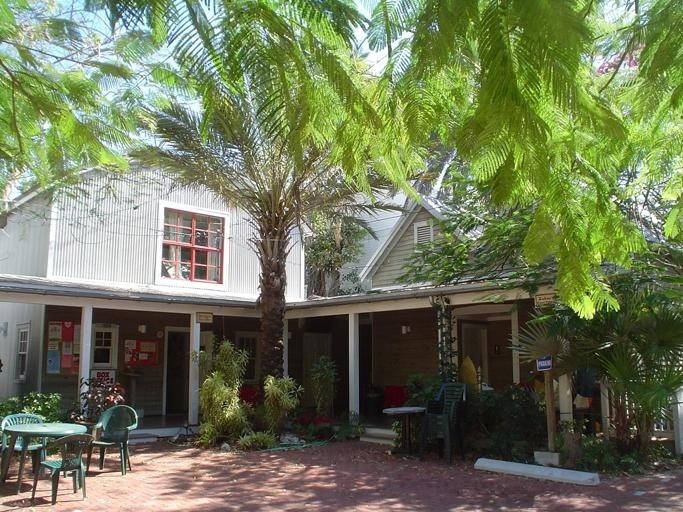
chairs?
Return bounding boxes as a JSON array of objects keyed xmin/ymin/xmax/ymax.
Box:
[
  {"xmin": 32, "ymin": 434, "xmax": 94, "ymax": 506},
  {"xmin": 419, "ymin": 382, "xmax": 465, "ymax": 465},
  {"xmin": 87, "ymin": 406, "xmax": 138, "ymax": 475},
  {"xmin": 1, "ymin": 414, "xmax": 46, "ymax": 479}
]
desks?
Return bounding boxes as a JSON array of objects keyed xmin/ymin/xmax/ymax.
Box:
[
  {"xmin": 382, "ymin": 406, "xmax": 426, "ymax": 459},
  {"xmin": 0, "ymin": 423, "xmax": 87, "ymax": 496}
]
